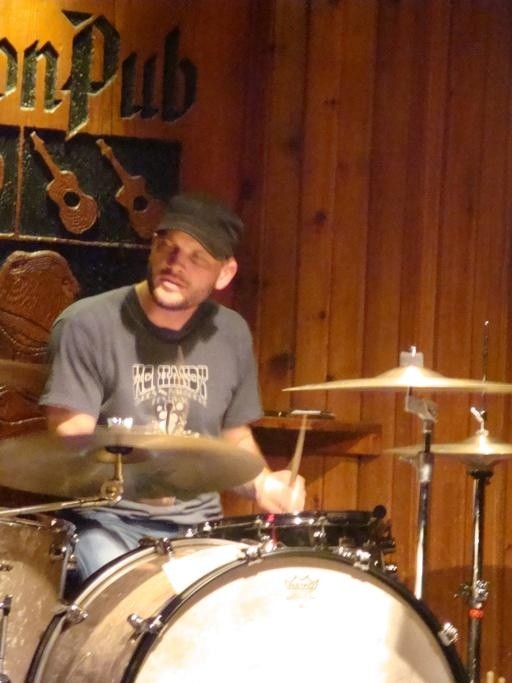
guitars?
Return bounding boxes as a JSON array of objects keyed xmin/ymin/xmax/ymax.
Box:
[{"xmin": 29, "ymin": 132, "xmax": 170, "ymax": 241}]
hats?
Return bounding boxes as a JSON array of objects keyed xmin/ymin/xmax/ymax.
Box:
[{"xmin": 153, "ymin": 193, "xmax": 245, "ymax": 260}]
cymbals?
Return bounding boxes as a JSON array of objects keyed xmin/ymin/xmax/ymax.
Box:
[
  {"xmin": 0, "ymin": 426, "xmax": 264, "ymax": 503},
  {"xmin": 384, "ymin": 436, "xmax": 512, "ymax": 467},
  {"xmin": 281, "ymin": 371, "xmax": 512, "ymax": 395}
]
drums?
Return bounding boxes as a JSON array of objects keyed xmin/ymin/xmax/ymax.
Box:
[
  {"xmin": 20, "ymin": 533, "xmax": 469, "ymax": 683},
  {"xmin": 185, "ymin": 504, "xmax": 398, "ymax": 578},
  {"xmin": 0, "ymin": 506, "xmax": 81, "ymax": 682}
]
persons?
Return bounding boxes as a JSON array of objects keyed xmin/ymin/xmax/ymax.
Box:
[{"xmin": 37, "ymin": 194, "xmax": 306, "ymax": 603}]
